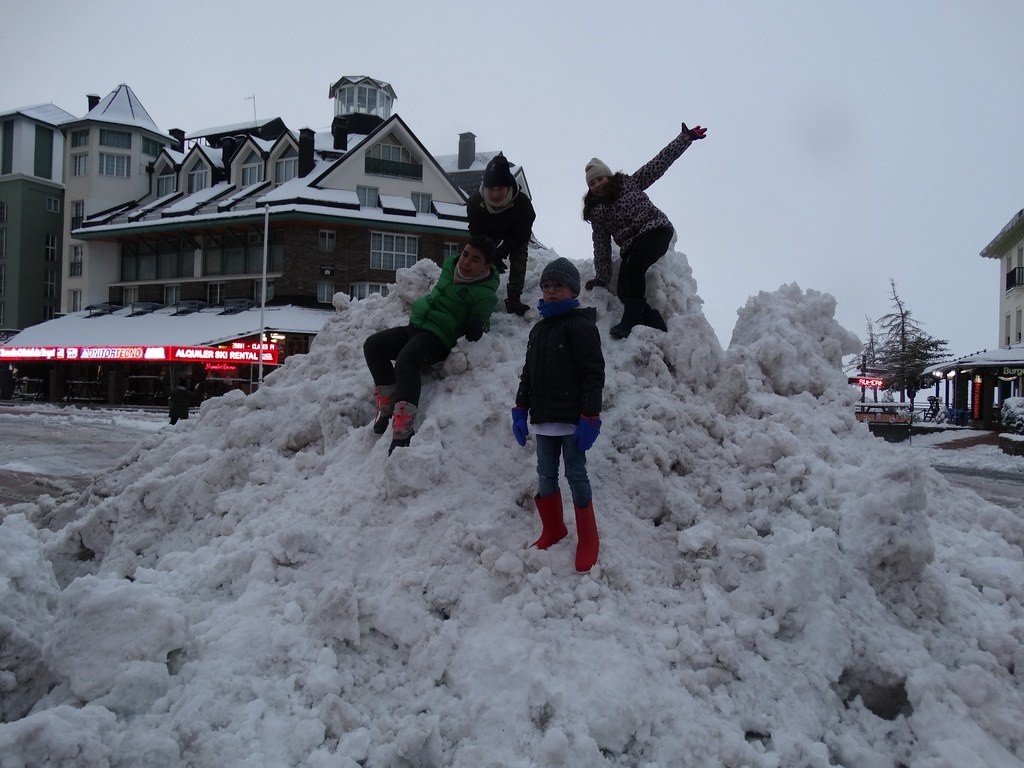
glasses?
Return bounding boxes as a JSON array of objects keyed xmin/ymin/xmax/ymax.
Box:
[{"xmin": 542, "ymin": 281, "xmax": 568, "ymax": 293}]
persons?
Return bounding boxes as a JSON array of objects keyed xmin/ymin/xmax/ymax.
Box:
[
  {"xmin": 467, "ymin": 155, "xmax": 536, "ymax": 316},
  {"xmin": 363, "ymin": 235, "xmax": 500, "ymax": 456},
  {"xmin": 583, "ymin": 123, "xmax": 707, "ymax": 337},
  {"xmin": 511, "ymin": 258, "xmax": 605, "ymax": 571},
  {"xmin": 168, "ymin": 379, "xmax": 194, "ymax": 425}
]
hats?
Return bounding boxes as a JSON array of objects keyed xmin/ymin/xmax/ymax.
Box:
[
  {"xmin": 585, "ymin": 158, "xmax": 613, "ymax": 186},
  {"xmin": 483, "ymin": 156, "xmax": 512, "ymax": 186},
  {"xmin": 540, "ymin": 258, "xmax": 581, "ymax": 299}
]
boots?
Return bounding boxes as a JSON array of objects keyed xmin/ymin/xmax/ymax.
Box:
[
  {"xmin": 643, "ymin": 304, "xmax": 668, "ymax": 333},
  {"xmin": 611, "ymin": 304, "xmax": 642, "ymax": 340},
  {"xmin": 388, "ymin": 401, "xmax": 417, "ymax": 456},
  {"xmin": 373, "ymin": 385, "xmax": 397, "ymax": 434},
  {"xmin": 574, "ymin": 500, "xmax": 599, "ymax": 574},
  {"xmin": 529, "ymin": 489, "xmax": 568, "ymax": 550}
]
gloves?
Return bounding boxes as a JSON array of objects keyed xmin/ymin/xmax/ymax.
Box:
[
  {"xmin": 507, "ymin": 292, "xmax": 529, "ymax": 317},
  {"xmin": 494, "ymin": 242, "xmax": 511, "ymax": 273},
  {"xmin": 511, "ymin": 407, "xmax": 528, "ymax": 447},
  {"xmin": 682, "ymin": 122, "xmax": 707, "ymax": 141},
  {"xmin": 575, "ymin": 414, "xmax": 602, "ymax": 451},
  {"xmin": 585, "ymin": 278, "xmax": 604, "ymax": 292},
  {"xmin": 465, "ymin": 320, "xmax": 485, "ymax": 342}
]
